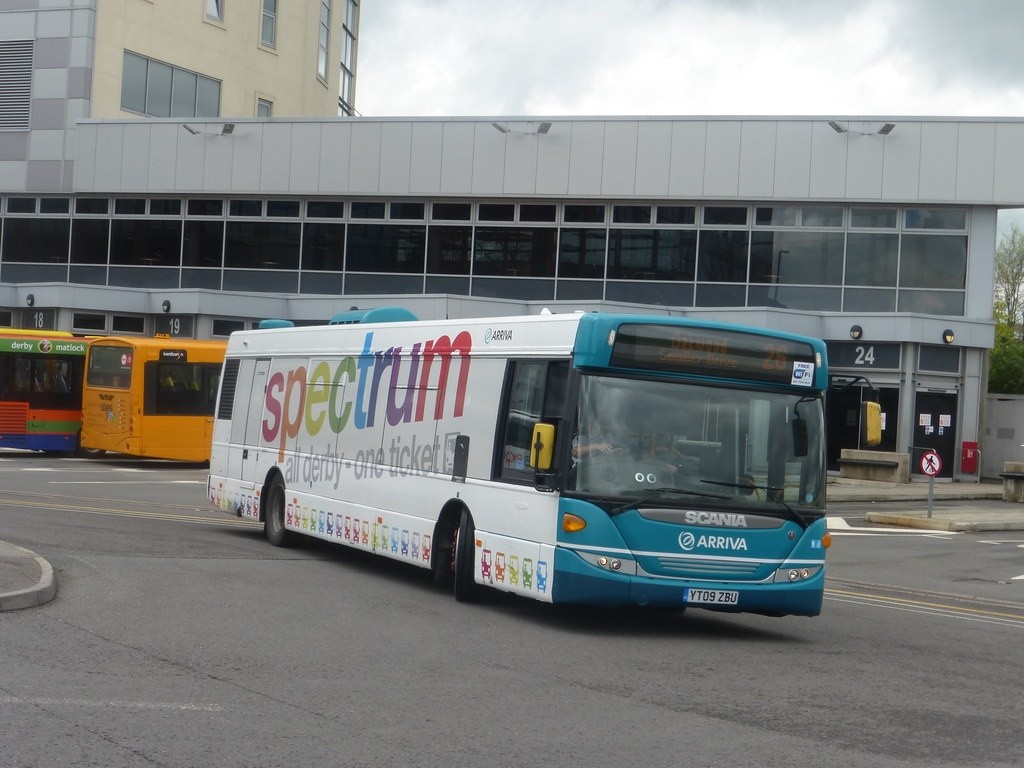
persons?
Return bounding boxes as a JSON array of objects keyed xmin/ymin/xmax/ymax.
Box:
[{"xmin": 570, "ymin": 387, "xmax": 631, "ymax": 469}]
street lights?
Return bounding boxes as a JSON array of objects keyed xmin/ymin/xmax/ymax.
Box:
[{"xmin": 773, "ymin": 250, "xmax": 791, "ymax": 301}]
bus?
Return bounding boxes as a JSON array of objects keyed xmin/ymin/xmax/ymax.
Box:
[
  {"xmin": 204, "ymin": 313, "xmax": 882, "ymax": 617},
  {"xmin": 80, "ymin": 332, "xmax": 228, "ymax": 464},
  {"xmin": 0, "ymin": 327, "xmax": 83, "ymax": 454}
]
